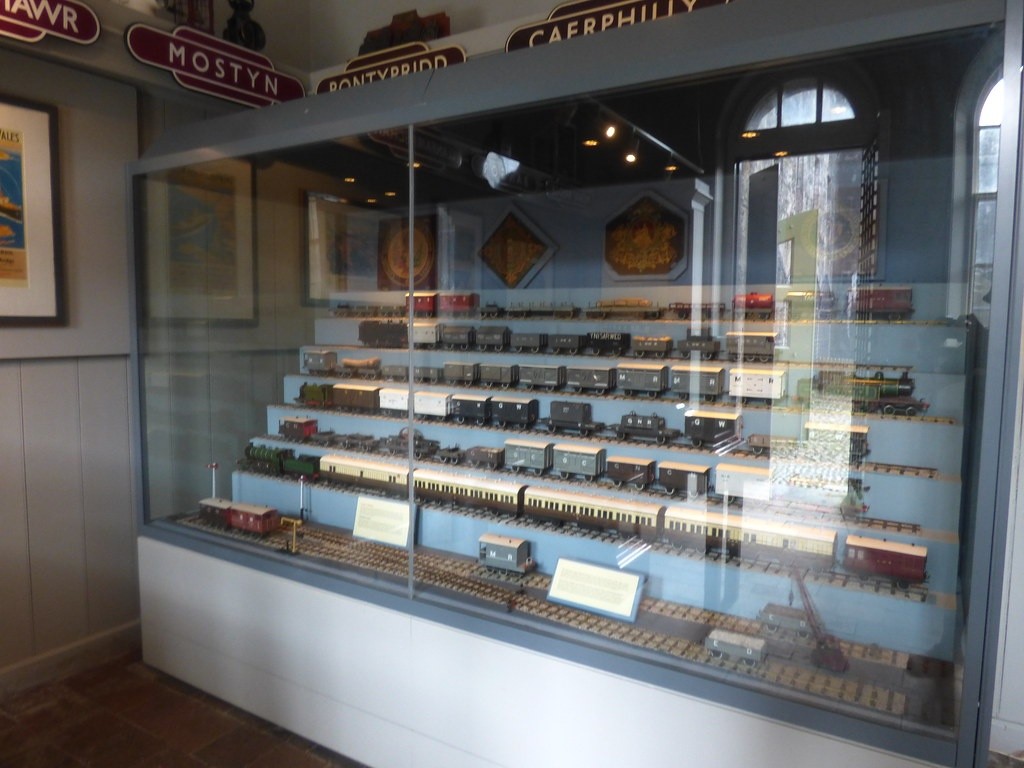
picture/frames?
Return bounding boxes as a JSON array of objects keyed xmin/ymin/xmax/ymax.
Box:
[
  {"xmin": 374, "ymin": 204, "xmax": 444, "ymax": 294},
  {"xmin": 143, "ymin": 153, "xmax": 260, "ymax": 327},
  {"xmin": 0, "ymin": 90, "xmax": 66, "ymax": 325},
  {"xmin": 298, "ymin": 188, "xmax": 381, "ymax": 309},
  {"xmin": 472, "ymin": 201, "xmax": 557, "ymax": 290}
]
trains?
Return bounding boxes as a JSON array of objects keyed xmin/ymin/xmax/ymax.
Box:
[{"xmin": 193, "ymin": 293, "xmax": 932, "ymax": 677}]
